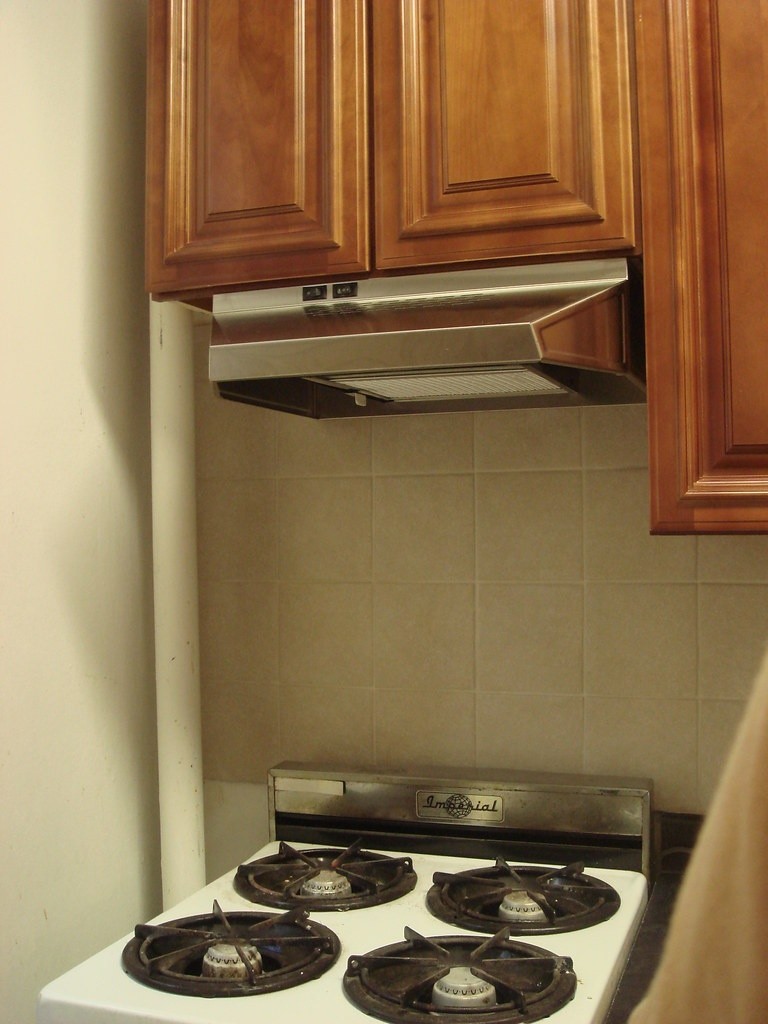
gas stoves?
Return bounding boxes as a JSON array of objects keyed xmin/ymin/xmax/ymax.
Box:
[{"xmin": 125, "ymin": 766, "xmax": 653, "ymax": 1024}]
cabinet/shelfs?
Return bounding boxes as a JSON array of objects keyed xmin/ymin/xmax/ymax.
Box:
[{"xmin": 145, "ymin": 0, "xmax": 768, "ymax": 542}]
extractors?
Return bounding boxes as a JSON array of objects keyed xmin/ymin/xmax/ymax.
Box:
[{"xmin": 208, "ymin": 257, "xmax": 644, "ymax": 421}]
